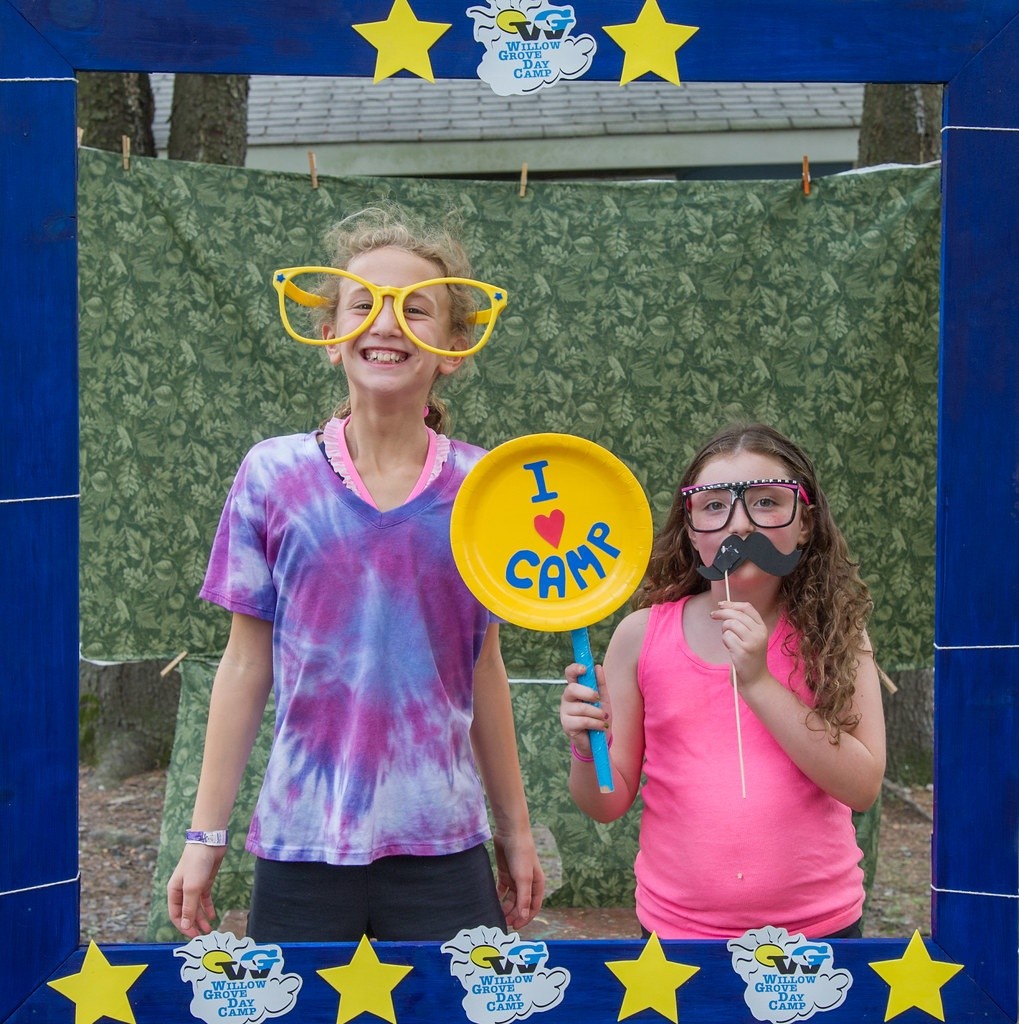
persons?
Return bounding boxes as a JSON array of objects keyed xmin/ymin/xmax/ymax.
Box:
[
  {"xmin": 560, "ymin": 423, "xmax": 886, "ymax": 939},
  {"xmin": 165, "ymin": 218, "xmax": 545, "ymax": 944}
]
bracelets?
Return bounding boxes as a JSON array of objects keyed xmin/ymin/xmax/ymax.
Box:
[
  {"xmin": 571, "ymin": 734, "xmax": 613, "ymax": 763},
  {"xmin": 183, "ymin": 829, "xmax": 230, "ymax": 848}
]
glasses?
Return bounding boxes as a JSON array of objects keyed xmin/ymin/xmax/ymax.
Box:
[
  {"xmin": 273, "ymin": 266, "xmax": 508, "ymax": 357},
  {"xmin": 680, "ymin": 479, "xmax": 810, "ymax": 533}
]
